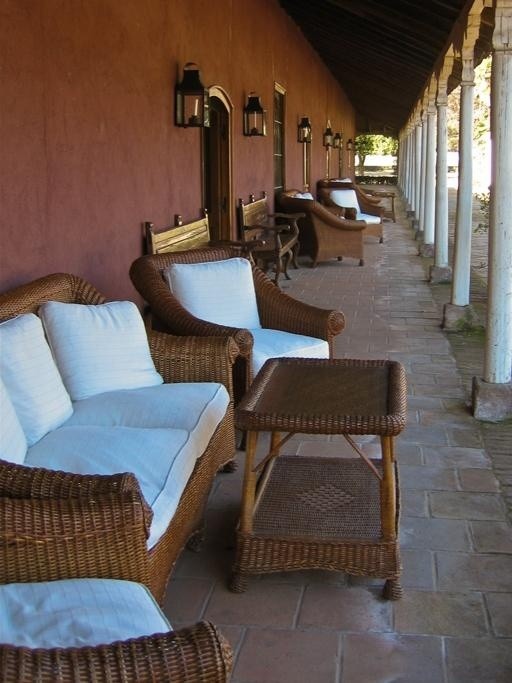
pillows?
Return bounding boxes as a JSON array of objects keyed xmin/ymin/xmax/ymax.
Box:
[
  {"xmin": 1, "ymin": 382, "xmax": 28, "ymax": 465},
  {"xmin": 0, "ymin": 313, "xmax": 73, "ymax": 448},
  {"xmin": 38, "ymin": 300, "xmax": 167, "ymax": 402},
  {"xmin": 163, "ymin": 257, "xmax": 261, "ymax": 331}
]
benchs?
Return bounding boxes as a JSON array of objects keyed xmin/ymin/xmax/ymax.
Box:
[
  {"xmin": 239, "ymin": 191, "xmax": 306, "ymax": 283},
  {"xmin": 144, "ymin": 207, "xmax": 265, "ymax": 264}
]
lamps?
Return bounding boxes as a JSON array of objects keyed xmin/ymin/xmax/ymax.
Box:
[
  {"xmin": 346, "ymin": 139, "xmax": 353, "ymax": 150},
  {"xmin": 297, "ymin": 116, "xmax": 312, "ymax": 143},
  {"xmin": 242, "ymin": 92, "xmax": 267, "ymax": 136},
  {"xmin": 354, "ymin": 142, "xmax": 358, "ymax": 150},
  {"xmin": 333, "ymin": 133, "xmax": 343, "ymax": 149},
  {"xmin": 323, "ymin": 128, "xmax": 335, "ymax": 146},
  {"xmin": 174, "ymin": 60, "xmax": 210, "ymax": 129}
]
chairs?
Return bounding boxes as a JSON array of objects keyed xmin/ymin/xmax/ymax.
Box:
[
  {"xmin": 276, "ymin": 177, "xmax": 385, "ymax": 268},
  {"xmin": 0, "ymin": 489, "xmax": 233, "ymax": 681},
  {"xmin": 129, "ymin": 245, "xmax": 347, "ymax": 451}
]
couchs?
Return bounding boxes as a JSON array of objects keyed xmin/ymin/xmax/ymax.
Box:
[{"xmin": 0, "ymin": 272, "xmax": 242, "ymax": 611}]
